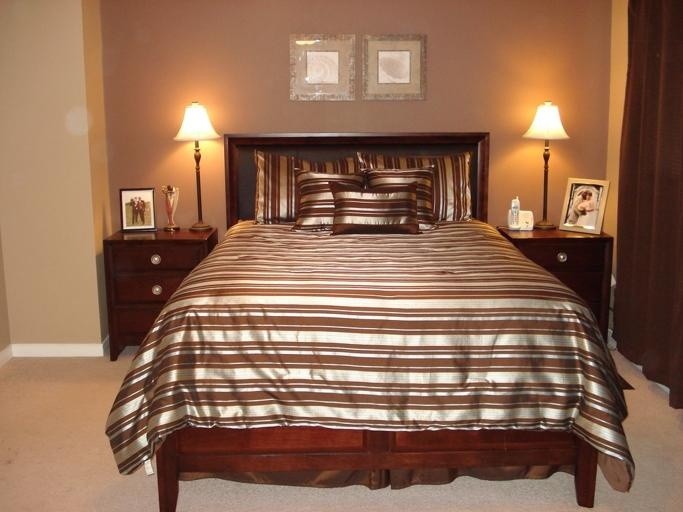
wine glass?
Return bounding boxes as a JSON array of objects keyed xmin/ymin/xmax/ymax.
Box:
[{"xmin": 159, "ymin": 185, "xmax": 179, "ymax": 230}]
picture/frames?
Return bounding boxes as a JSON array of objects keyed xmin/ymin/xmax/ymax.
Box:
[
  {"xmin": 288, "ymin": 32, "xmax": 356, "ymax": 102},
  {"xmin": 118, "ymin": 188, "xmax": 156, "ymax": 232},
  {"xmin": 359, "ymin": 32, "xmax": 426, "ymax": 101},
  {"xmin": 557, "ymin": 177, "xmax": 610, "ymax": 235}
]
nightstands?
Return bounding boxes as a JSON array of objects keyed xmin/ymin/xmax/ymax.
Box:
[{"xmin": 494, "ymin": 222, "xmax": 613, "ymax": 349}]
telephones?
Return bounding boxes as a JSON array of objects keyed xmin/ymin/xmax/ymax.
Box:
[{"xmin": 507, "ymin": 196, "xmax": 534, "ymax": 231}]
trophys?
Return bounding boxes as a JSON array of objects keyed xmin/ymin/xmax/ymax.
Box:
[{"xmin": 162, "ymin": 185, "xmax": 180, "ymax": 232}]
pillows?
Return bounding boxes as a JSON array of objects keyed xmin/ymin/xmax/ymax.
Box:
[
  {"xmin": 250, "ymin": 147, "xmax": 362, "ymax": 226},
  {"xmin": 353, "ymin": 150, "xmax": 475, "ymax": 222},
  {"xmin": 363, "ymin": 165, "xmax": 440, "ymax": 231},
  {"xmin": 330, "ymin": 181, "xmax": 423, "ymax": 237},
  {"xmin": 289, "ymin": 166, "xmax": 365, "ymax": 231}
]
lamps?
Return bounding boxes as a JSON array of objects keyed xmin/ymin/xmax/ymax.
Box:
[
  {"xmin": 173, "ymin": 101, "xmax": 220, "ymax": 232},
  {"xmin": 522, "ymin": 100, "xmax": 569, "ymax": 231}
]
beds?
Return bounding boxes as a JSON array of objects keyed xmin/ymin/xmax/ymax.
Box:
[{"xmin": 103, "ymin": 131, "xmax": 634, "ymax": 512}]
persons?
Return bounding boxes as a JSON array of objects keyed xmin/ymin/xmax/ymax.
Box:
[
  {"xmin": 130, "ymin": 196, "xmax": 146, "ymax": 225},
  {"xmin": 576, "ymin": 190, "xmax": 597, "ymax": 226}
]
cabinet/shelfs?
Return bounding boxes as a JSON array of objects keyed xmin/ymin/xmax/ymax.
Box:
[{"xmin": 102, "ymin": 226, "xmax": 217, "ymax": 361}]
